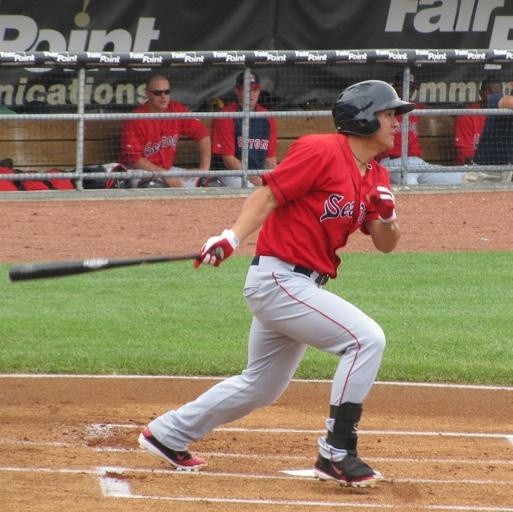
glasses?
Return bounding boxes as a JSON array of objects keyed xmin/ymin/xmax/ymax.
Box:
[{"xmin": 146, "ymin": 87, "xmax": 172, "ymax": 97}]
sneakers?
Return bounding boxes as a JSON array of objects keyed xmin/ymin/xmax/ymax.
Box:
[
  {"xmin": 138, "ymin": 425, "xmax": 208, "ymax": 471},
  {"xmin": 314, "ymin": 452, "xmax": 383, "ymax": 489}
]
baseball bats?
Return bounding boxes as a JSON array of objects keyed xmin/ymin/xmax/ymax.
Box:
[{"xmin": 9, "ymin": 246, "xmax": 226, "ymax": 281}]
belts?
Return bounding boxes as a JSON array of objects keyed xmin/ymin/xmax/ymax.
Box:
[{"xmin": 250, "ymin": 255, "xmax": 330, "ymax": 286}]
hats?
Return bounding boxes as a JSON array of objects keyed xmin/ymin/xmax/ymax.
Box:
[
  {"xmin": 393, "ymin": 71, "xmax": 420, "ymax": 90},
  {"xmin": 236, "ymin": 71, "xmax": 264, "ymax": 90}
]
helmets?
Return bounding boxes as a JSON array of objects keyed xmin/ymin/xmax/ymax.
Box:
[{"xmin": 331, "ymin": 80, "xmax": 416, "ymax": 137}]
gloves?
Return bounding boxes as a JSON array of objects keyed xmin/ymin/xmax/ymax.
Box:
[
  {"xmin": 368, "ymin": 181, "xmax": 397, "ymax": 224},
  {"xmin": 193, "ymin": 227, "xmax": 241, "ymax": 271}
]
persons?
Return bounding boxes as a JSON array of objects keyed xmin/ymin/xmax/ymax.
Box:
[
  {"xmin": 116, "ymin": 74, "xmax": 224, "ymax": 188},
  {"xmin": 372, "ymin": 70, "xmax": 464, "ymax": 187},
  {"xmin": 452, "ymin": 77, "xmax": 502, "ymax": 166},
  {"xmin": 136, "ymin": 79, "xmax": 417, "ymax": 489},
  {"xmin": 463, "ymin": 72, "xmax": 513, "ymax": 189},
  {"xmin": 211, "ymin": 70, "xmax": 277, "ymax": 188}
]
